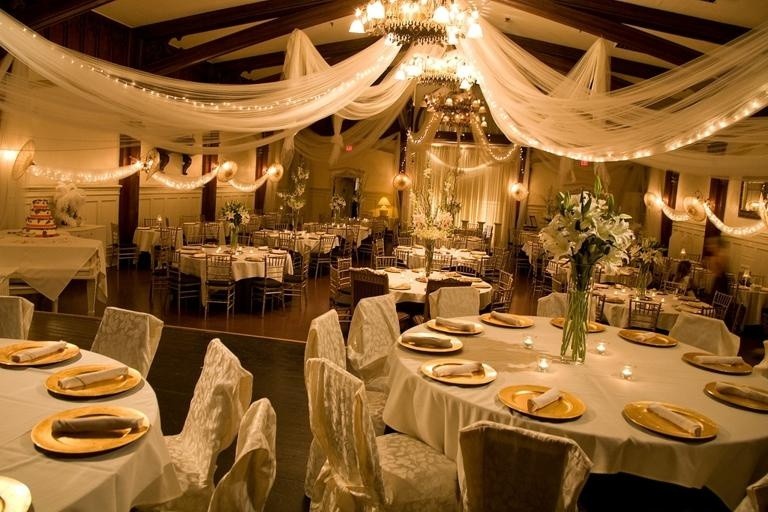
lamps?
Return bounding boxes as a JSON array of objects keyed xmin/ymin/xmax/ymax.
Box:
[
  {"xmin": 392, "ymin": 140, "xmax": 411, "ymax": 190},
  {"xmin": 643, "ymin": 191, "xmax": 768, "ymax": 236},
  {"xmin": 349, "ymin": 0, "xmax": 487, "ymax": 128},
  {"xmin": 508, "ymin": 147, "xmax": 529, "ymax": 202},
  {"xmin": 10, "ymin": 140, "xmax": 283, "ymax": 192},
  {"xmin": 376, "ymin": 196, "xmax": 391, "ymax": 216}
]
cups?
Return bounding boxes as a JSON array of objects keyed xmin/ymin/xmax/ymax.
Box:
[
  {"xmin": 595, "ymin": 339, "xmax": 612, "ymax": 355},
  {"xmin": 521, "ymin": 333, "xmax": 538, "ymax": 350},
  {"xmin": 536, "ymin": 353, "xmax": 553, "ymax": 373},
  {"xmin": 618, "ymin": 361, "xmax": 637, "ymax": 382}
]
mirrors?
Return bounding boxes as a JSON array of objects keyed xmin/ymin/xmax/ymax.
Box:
[{"xmin": 738, "ymin": 176, "xmax": 768, "ymax": 219}]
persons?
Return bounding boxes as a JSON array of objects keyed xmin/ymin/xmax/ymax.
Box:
[
  {"xmin": 700, "ymin": 235, "xmax": 734, "ymax": 330},
  {"xmin": 671, "ymin": 260, "xmax": 692, "ymax": 296}
]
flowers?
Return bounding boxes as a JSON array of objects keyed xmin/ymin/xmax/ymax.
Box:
[
  {"xmin": 277, "ymin": 161, "xmax": 311, "ymax": 212},
  {"xmin": 52, "ymin": 184, "xmax": 87, "ymax": 226},
  {"xmin": 329, "ymin": 193, "xmax": 346, "ymax": 212}
]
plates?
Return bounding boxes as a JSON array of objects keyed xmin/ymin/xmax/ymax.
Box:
[
  {"xmin": 398, "ymin": 319, "xmax": 497, "ymax": 386},
  {"xmin": 254, "ymin": 228, "xmax": 336, "ymax": 240},
  {"xmin": 396, "ymin": 246, "xmax": 489, "ymax": 260},
  {"xmin": 706, "ymin": 381, "xmax": 768, "ymax": 412},
  {"xmin": 549, "ymin": 316, "xmax": 606, "ymax": 333},
  {"xmin": 497, "ymin": 384, "xmax": 586, "ymax": 420},
  {"xmin": 591, "ymin": 282, "xmax": 715, "ymax": 315},
  {"xmin": 681, "ymin": 351, "xmax": 754, "ymax": 375},
  {"xmin": 137, "ymin": 226, "xmax": 182, "ymax": 232},
  {"xmin": 479, "ymin": 314, "xmax": 535, "ymax": 328},
  {"xmin": 30, "ymin": 406, "xmax": 149, "ymax": 456},
  {"xmin": 176, "ymin": 242, "xmax": 287, "ymax": 262},
  {"xmin": 622, "ymin": 401, "xmax": 723, "ymax": 441},
  {"xmin": 739, "ymin": 287, "xmax": 768, "ymax": 292},
  {"xmin": 45, "ymin": 365, "xmax": 141, "ymax": 399},
  {"xmin": 1, "ymin": 476, "xmax": 34, "ymax": 512},
  {"xmin": 382, "ymin": 266, "xmax": 491, "ymax": 291},
  {"xmin": 618, "ymin": 328, "xmax": 679, "ymax": 347},
  {"xmin": 0, "ymin": 340, "xmax": 81, "ymax": 368}
]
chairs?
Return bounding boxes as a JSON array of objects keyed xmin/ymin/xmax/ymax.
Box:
[{"xmin": 3, "ymin": 212, "xmax": 766, "ymax": 507}]
